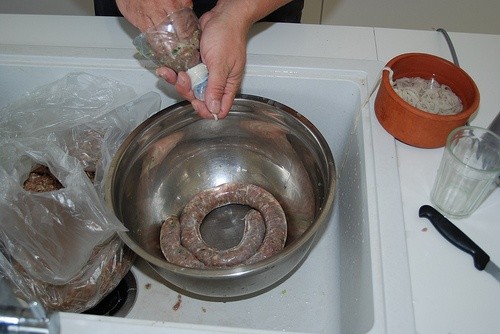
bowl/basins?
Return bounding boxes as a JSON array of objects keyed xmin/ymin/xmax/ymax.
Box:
[
  {"xmin": 374, "ymin": 53, "xmax": 481, "ymax": 150},
  {"xmin": 104, "ymin": 94, "xmax": 337, "ymax": 297}
]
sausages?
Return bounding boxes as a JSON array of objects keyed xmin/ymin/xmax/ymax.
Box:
[{"xmin": 160, "ymin": 182, "xmax": 287, "ymax": 270}]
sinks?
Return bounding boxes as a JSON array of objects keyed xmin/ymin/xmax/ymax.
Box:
[{"xmin": 1, "ymin": 46, "xmax": 418, "ymax": 334}]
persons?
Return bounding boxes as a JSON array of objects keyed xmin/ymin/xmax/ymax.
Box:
[{"xmin": 93, "ymin": 0, "xmax": 305, "ymax": 119}]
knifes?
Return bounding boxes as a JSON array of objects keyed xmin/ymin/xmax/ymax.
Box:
[{"xmin": 419, "ymin": 204, "xmax": 500, "ymax": 283}]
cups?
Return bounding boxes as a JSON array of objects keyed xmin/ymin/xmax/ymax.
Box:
[{"xmin": 431, "ymin": 125, "xmax": 500, "ymax": 217}]
